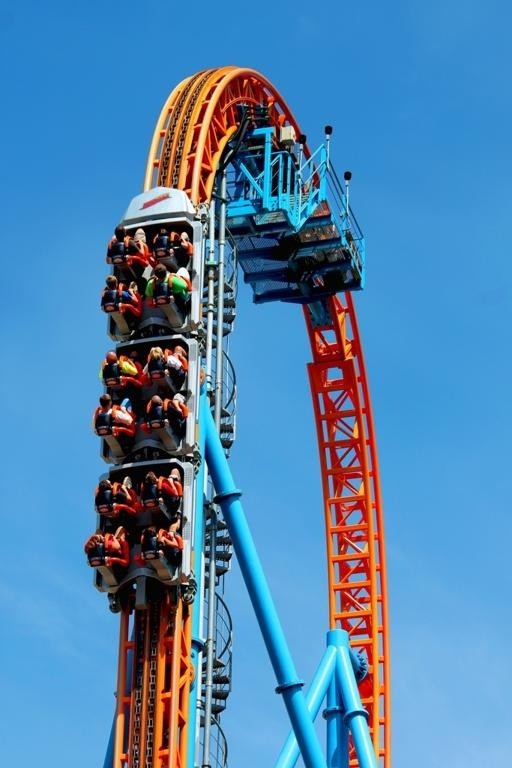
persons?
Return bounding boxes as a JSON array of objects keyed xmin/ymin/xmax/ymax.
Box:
[
  {"xmin": 85, "ymin": 468, "xmax": 183, "ymax": 557},
  {"xmin": 93, "ymin": 345, "xmax": 188, "ymax": 431},
  {"xmin": 100, "ymin": 224, "xmax": 192, "ymax": 304}
]
